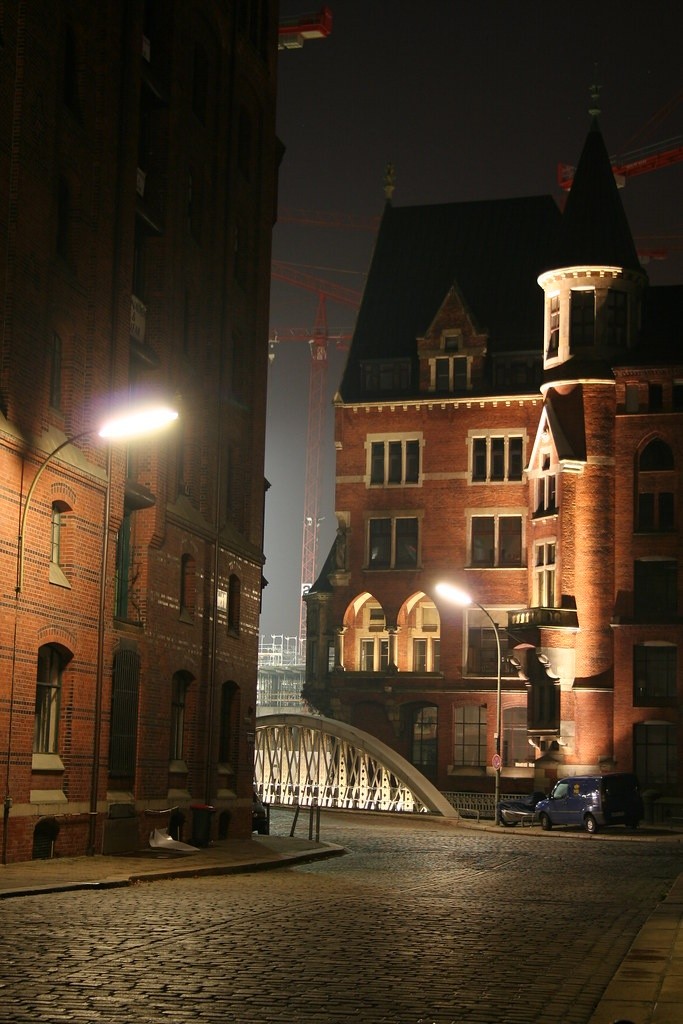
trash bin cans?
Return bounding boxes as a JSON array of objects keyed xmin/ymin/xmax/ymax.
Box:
[{"xmin": 190, "ymin": 804, "xmax": 216, "ymax": 846}]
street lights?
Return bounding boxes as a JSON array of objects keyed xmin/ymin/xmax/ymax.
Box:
[{"xmin": 436, "ymin": 581, "xmax": 502, "ymax": 831}]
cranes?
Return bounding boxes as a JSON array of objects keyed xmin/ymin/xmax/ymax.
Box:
[
  {"xmin": 261, "ymin": 201, "xmax": 383, "ymax": 660},
  {"xmin": 556, "ymin": 131, "xmax": 682, "ymax": 201}
]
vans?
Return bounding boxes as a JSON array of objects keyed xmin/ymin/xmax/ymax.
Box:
[{"xmin": 536, "ymin": 771, "xmax": 644, "ymax": 835}]
